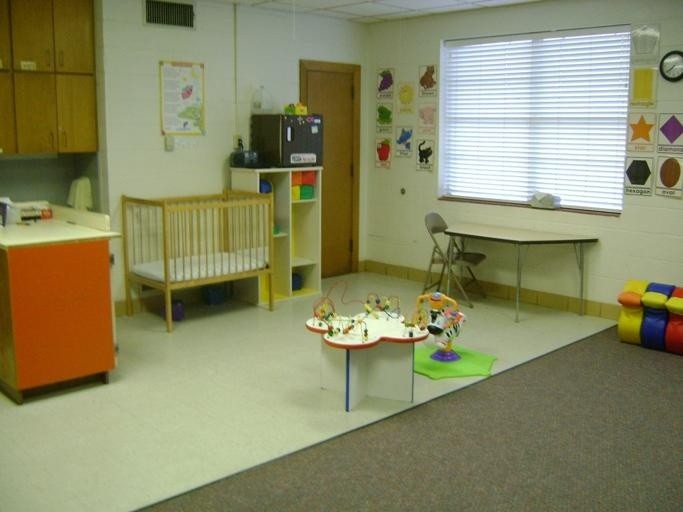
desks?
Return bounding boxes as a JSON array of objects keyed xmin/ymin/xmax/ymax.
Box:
[{"xmin": 441, "ymin": 223, "xmax": 598, "ymax": 322}]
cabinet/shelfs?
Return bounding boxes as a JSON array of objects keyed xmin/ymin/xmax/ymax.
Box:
[
  {"xmin": 1, "ymin": 0, "xmax": 99, "ymax": 156},
  {"xmin": 231, "ymin": 160, "xmax": 324, "ymax": 308}
]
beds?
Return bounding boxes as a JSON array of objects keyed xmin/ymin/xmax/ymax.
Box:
[{"xmin": 119, "ymin": 190, "xmax": 276, "ymax": 334}]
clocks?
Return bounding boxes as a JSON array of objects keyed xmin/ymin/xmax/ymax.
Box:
[{"xmin": 659, "ymin": 49, "xmax": 683, "ymax": 81}]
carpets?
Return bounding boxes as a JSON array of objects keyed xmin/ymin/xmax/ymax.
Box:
[{"xmin": 134, "ymin": 322, "xmax": 683, "ymax": 511}]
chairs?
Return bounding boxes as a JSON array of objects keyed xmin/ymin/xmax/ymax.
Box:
[{"xmin": 421, "ymin": 213, "xmax": 485, "ymax": 309}]
